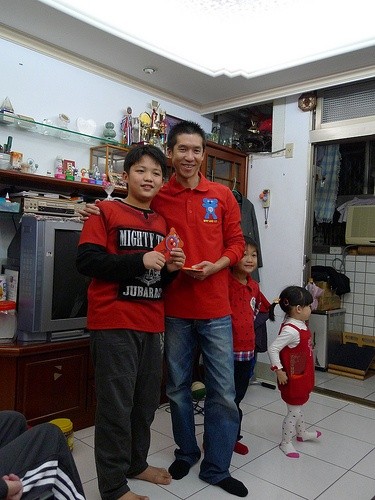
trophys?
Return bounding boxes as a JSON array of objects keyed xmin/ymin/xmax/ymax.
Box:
[{"xmin": 119, "ymin": 98, "xmax": 168, "ymax": 149}]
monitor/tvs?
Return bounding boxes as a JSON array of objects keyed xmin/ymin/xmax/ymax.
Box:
[{"xmin": 7, "ymin": 213, "xmax": 92, "ymax": 341}]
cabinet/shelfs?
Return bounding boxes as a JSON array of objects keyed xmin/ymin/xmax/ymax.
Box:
[
  {"xmin": 0, "ymin": 343, "xmax": 166, "ymax": 433},
  {"xmin": 1, "ymin": 110, "xmax": 134, "ymax": 195},
  {"xmin": 167, "ymin": 140, "xmax": 249, "ymax": 402}
]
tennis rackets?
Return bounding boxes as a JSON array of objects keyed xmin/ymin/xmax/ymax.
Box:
[{"xmin": 250, "ymin": 377, "xmax": 276, "ymax": 390}]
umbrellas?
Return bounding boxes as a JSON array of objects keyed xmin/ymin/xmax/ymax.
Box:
[{"xmin": 305, "ymin": 278, "xmax": 325, "ymax": 311}]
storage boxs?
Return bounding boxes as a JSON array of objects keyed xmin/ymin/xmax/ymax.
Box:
[{"xmin": 305, "ymin": 282, "xmax": 346, "ymax": 370}]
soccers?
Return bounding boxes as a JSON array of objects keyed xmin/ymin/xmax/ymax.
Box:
[{"xmin": 192, "ymin": 382, "xmax": 206, "ymax": 398}]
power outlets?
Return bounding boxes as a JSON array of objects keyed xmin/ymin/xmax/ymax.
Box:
[{"xmin": 285, "ymin": 143, "xmax": 294, "ymax": 158}]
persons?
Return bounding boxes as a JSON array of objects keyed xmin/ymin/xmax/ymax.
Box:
[
  {"xmin": 268, "ymin": 285, "xmax": 325, "ymax": 459},
  {"xmin": 77, "ymin": 122, "xmax": 250, "ymax": 497},
  {"xmin": 0, "ymin": 408, "xmax": 89, "ymax": 500},
  {"xmin": 78, "ymin": 143, "xmax": 187, "ymax": 500},
  {"xmin": 224, "ymin": 234, "xmax": 271, "ymax": 455}
]
